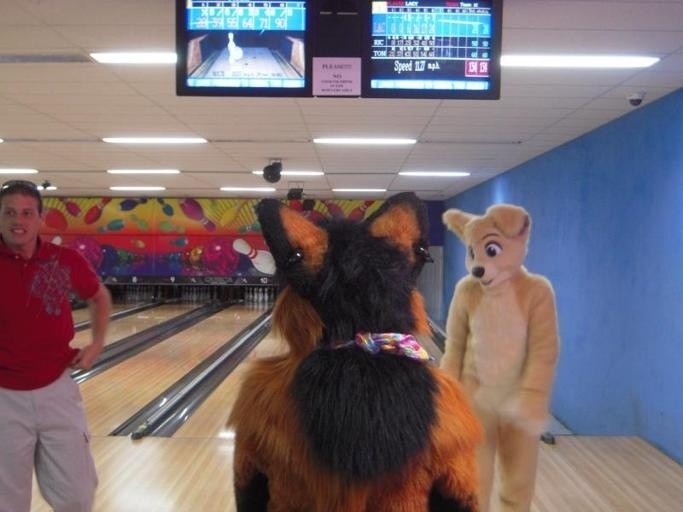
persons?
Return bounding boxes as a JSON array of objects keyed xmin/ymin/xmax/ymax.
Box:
[{"xmin": 0, "ymin": 179, "xmax": 112, "ymax": 511}]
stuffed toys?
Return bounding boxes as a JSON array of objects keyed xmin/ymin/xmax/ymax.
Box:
[
  {"xmin": 438, "ymin": 204, "xmax": 561, "ymax": 511},
  {"xmin": 223, "ymin": 190, "xmax": 488, "ymax": 512}
]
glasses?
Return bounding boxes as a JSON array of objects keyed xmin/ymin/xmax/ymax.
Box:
[{"xmin": 3, "ymin": 178, "xmax": 37, "ymax": 190}]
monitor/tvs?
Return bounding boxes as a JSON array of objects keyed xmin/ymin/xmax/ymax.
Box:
[
  {"xmin": 360, "ymin": 0, "xmax": 503, "ymax": 100},
  {"xmin": 174, "ymin": 0, "xmax": 314, "ymax": 98}
]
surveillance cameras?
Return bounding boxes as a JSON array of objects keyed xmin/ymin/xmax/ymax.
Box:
[{"xmin": 627, "ymin": 93, "xmax": 644, "ymax": 107}]
